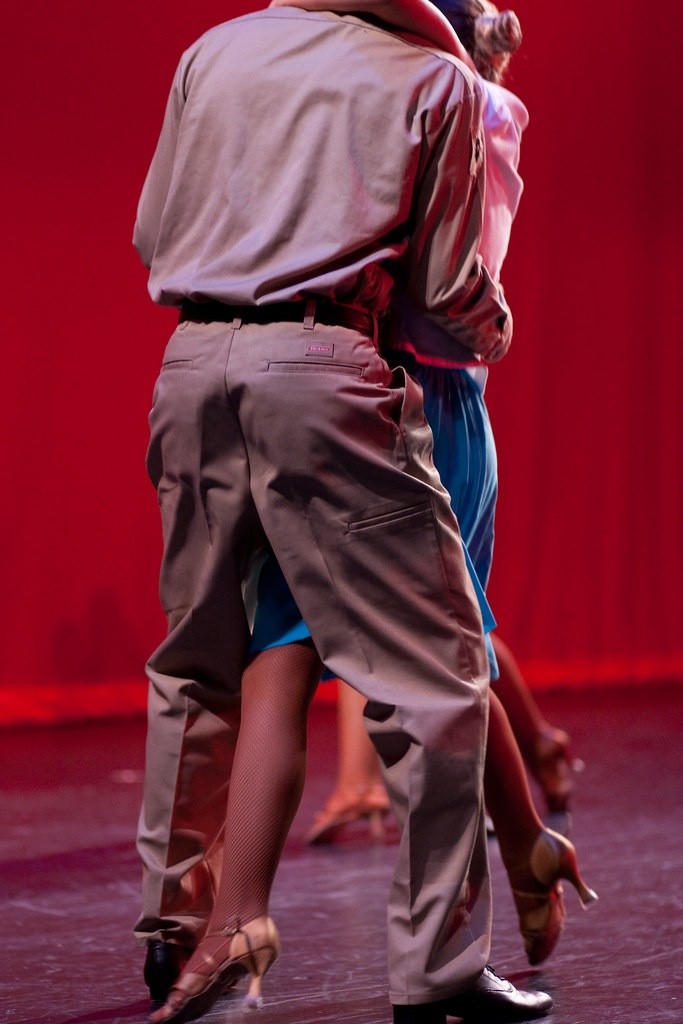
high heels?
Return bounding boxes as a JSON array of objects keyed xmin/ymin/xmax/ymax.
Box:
[
  {"xmin": 509, "ymin": 827, "xmax": 600, "ymax": 967},
  {"xmin": 303, "ymin": 787, "xmax": 392, "ymax": 846},
  {"xmin": 147, "ymin": 914, "xmax": 283, "ymax": 1024},
  {"xmin": 532, "ymin": 725, "xmax": 586, "ymax": 815}
]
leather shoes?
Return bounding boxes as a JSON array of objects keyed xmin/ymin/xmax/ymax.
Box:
[
  {"xmin": 390, "ymin": 964, "xmax": 553, "ymax": 1024},
  {"xmin": 142, "ymin": 939, "xmax": 248, "ymax": 1007}
]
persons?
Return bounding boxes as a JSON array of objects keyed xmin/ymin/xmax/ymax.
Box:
[
  {"xmin": 133, "ymin": 0, "xmax": 594, "ymax": 1024},
  {"xmin": 310, "ymin": 633, "xmax": 583, "ymax": 846}
]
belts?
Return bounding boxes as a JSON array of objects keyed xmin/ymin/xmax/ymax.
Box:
[{"xmin": 176, "ymin": 297, "xmax": 374, "ymax": 338}]
